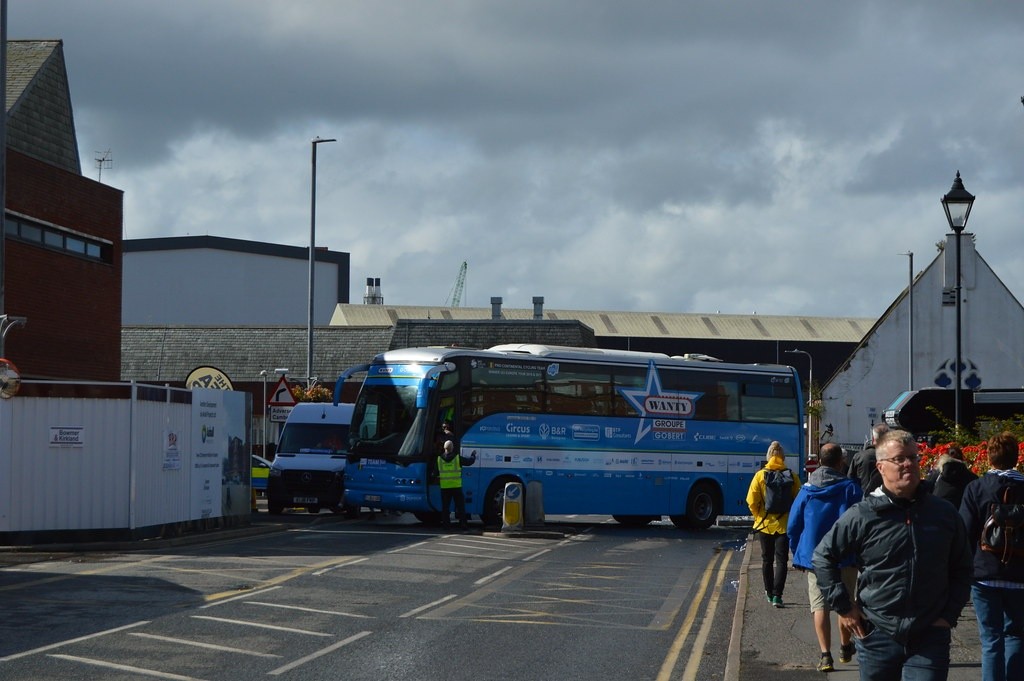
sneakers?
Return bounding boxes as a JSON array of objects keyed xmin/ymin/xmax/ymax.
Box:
[
  {"xmin": 817, "ymin": 656, "xmax": 834, "ymax": 671},
  {"xmin": 839, "ymin": 641, "xmax": 857, "ymax": 663},
  {"xmin": 768, "ymin": 596, "xmax": 774, "ymax": 603},
  {"xmin": 773, "ymin": 596, "xmax": 785, "ymax": 608}
]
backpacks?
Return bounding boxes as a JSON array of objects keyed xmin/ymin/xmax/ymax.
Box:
[
  {"xmin": 980, "ymin": 473, "xmax": 1024, "ymax": 563},
  {"xmin": 761, "ymin": 467, "xmax": 794, "ymax": 514}
]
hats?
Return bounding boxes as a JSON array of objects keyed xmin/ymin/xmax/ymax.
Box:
[{"xmin": 767, "ymin": 440, "xmax": 785, "ymax": 461}]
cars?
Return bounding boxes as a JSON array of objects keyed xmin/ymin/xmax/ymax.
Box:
[{"xmin": 251, "ymin": 453, "xmax": 272, "ymax": 493}]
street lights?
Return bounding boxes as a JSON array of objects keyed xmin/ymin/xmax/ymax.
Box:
[
  {"xmin": 939, "ymin": 169, "xmax": 976, "ymax": 447},
  {"xmin": 303, "ymin": 135, "xmax": 339, "ymax": 396},
  {"xmin": 784, "ymin": 349, "xmax": 811, "ymax": 483},
  {"xmin": 895, "ymin": 251, "xmax": 914, "ymax": 393}
]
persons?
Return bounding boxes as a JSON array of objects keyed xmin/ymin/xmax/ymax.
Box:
[
  {"xmin": 304, "ymin": 432, "xmax": 322, "ymax": 448},
  {"xmin": 745, "ymin": 441, "xmax": 801, "ymax": 606},
  {"xmin": 323, "ymin": 432, "xmax": 342, "ymax": 449},
  {"xmin": 923, "ymin": 447, "xmax": 980, "ymax": 511},
  {"xmin": 432, "ymin": 440, "xmax": 476, "ymax": 532},
  {"xmin": 841, "ymin": 447, "xmax": 849, "ymax": 474},
  {"xmin": 440, "ymin": 419, "xmax": 455, "ymax": 444},
  {"xmin": 847, "ymin": 423, "xmax": 890, "ymax": 492},
  {"xmin": 958, "ymin": 430, "xmax": 1024, "ymax": 681},
  {"xmin": 812, "ymin": 430, "xmax": 975, "ymax": 681},
  {"xmin": 786, "ymin": 442, "xmax": 864, "ymax": 670}
]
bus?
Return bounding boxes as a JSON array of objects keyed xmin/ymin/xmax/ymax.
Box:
[{"xmin": 332, "ymin": 342, "xmax": 807, "ymax": 529}]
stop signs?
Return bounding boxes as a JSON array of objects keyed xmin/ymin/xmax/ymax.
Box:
[{"xmin": 804, "ymin": 459, "xmax": 817, "ymax": 473}]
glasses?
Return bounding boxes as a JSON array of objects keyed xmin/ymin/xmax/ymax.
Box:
[{"xmin": 880, "ymin": 453, "xmax": 921, "ymax": 464}]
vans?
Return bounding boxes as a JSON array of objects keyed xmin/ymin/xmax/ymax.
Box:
[{"xmin": 266, "ymin": 401, "xmax": 379, "ymax": 515}]
